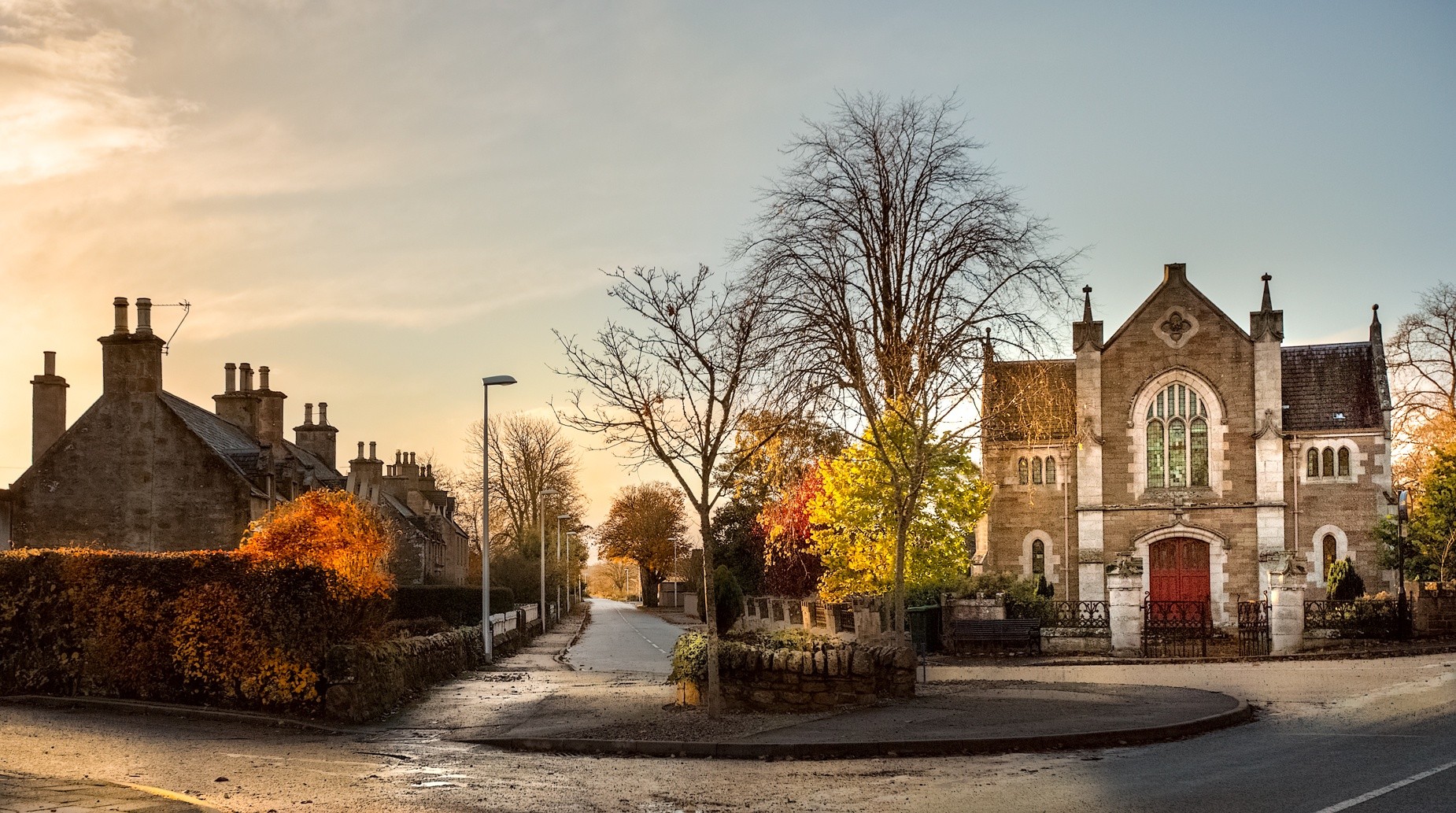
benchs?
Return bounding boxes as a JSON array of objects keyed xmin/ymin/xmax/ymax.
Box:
[{"xmin": 954, "ymin": 619, "xmax": 1043, "ymax": 658}]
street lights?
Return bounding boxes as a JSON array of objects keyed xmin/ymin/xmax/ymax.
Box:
[
  {"xmin": 666, "ymin": 537, "xmax": 677, "ymax": 608},
  {"xmin": 557, "ymin": 513, "xmax": 572, "ymax": 620},
  {"xmin": 623, "ymin": 568, "xmax": 629, "ymax": 599},
  {"xmin": 480, "ymin": 375, "xmax": 518, "ymax": 662},
  {"xmin": 566, "ymin": 531, "xmax": 578, "ymax": 616},
  {"xmin": 585, "ymin": 573, "xmax": 588, "ymax": 585},
  {"xmin": 540, "ymin": 489, "xmax": 559, "ymax": 631}
]
